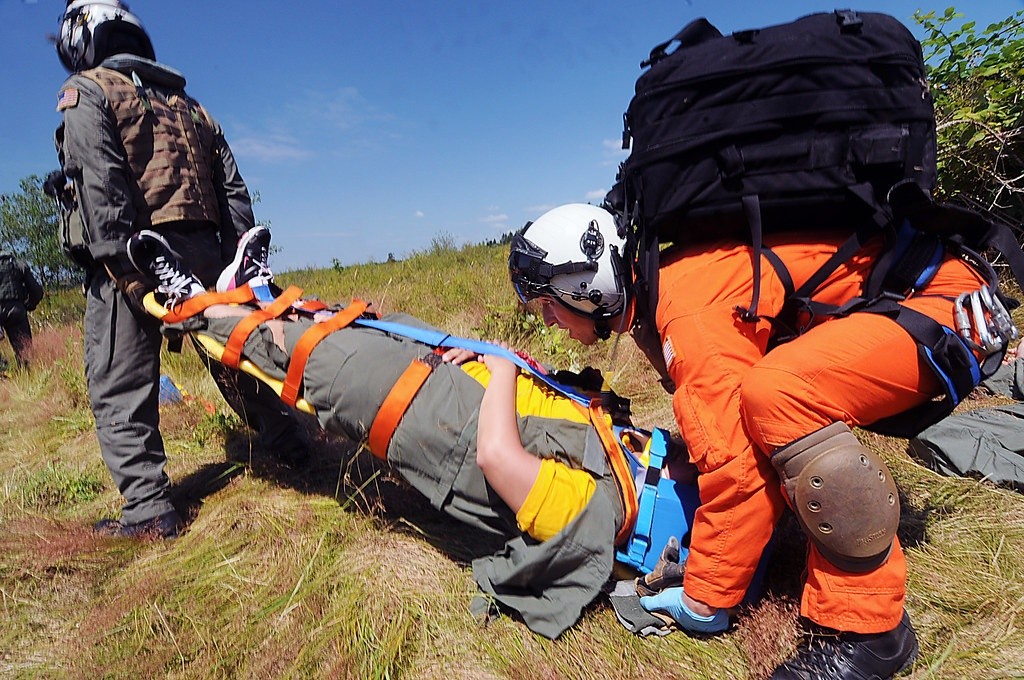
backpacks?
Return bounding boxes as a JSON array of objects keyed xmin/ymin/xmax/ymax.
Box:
[{"xmin": 604, "ymin": 9, "xmax": 1024, "ymax": 393}]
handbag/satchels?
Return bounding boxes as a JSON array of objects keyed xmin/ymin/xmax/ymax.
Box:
[{"xmin": 9, "ymin": 254, "xmax": 43, "ymax": 311}]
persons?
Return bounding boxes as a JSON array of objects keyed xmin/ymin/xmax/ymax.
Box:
[
  {"xmin": 0, "ymin": 244, "xmax": 44, "ymax": 377},
  {"xmin": 125, "ymin": 225, "xmax": 785, "ymax": 607},
  {"xmin": 507, "ymin": 203, "xmax": 1010, "ymax": 680},
  {"xmin": 46, "ymin": 0, "xmax": 311, "ymax": 540}
]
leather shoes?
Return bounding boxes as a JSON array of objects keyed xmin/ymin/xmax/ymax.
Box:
[{"xmin": 770, "ymin": 608, "xmax": 917, "ymax": 680}]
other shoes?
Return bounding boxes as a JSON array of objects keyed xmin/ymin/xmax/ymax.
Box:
[{"xmin": 95, "ymin": 510, "xmax": 184, "ymax": 540}]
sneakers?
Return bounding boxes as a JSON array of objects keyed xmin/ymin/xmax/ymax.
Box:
[
  {"xmin": 126, "ymin": 229, "xmax": 205, "ymax": 311},
  {"xmin": 215, "ymin": 225, "xmax": 272, "ymax": 294}
]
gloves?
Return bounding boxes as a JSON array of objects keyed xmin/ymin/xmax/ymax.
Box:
[{"xmin": 639, "ymin": 587, "xmax": 729, "ymax": 633}]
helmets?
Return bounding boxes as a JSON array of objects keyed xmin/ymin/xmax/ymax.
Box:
[
  {"xmin": 517, "ymin": 203, "xmax": 627, "ymax": 317},
  {"xmin": 56, "ymin": 0, "xmax": 157, "ymax": 72}
]
